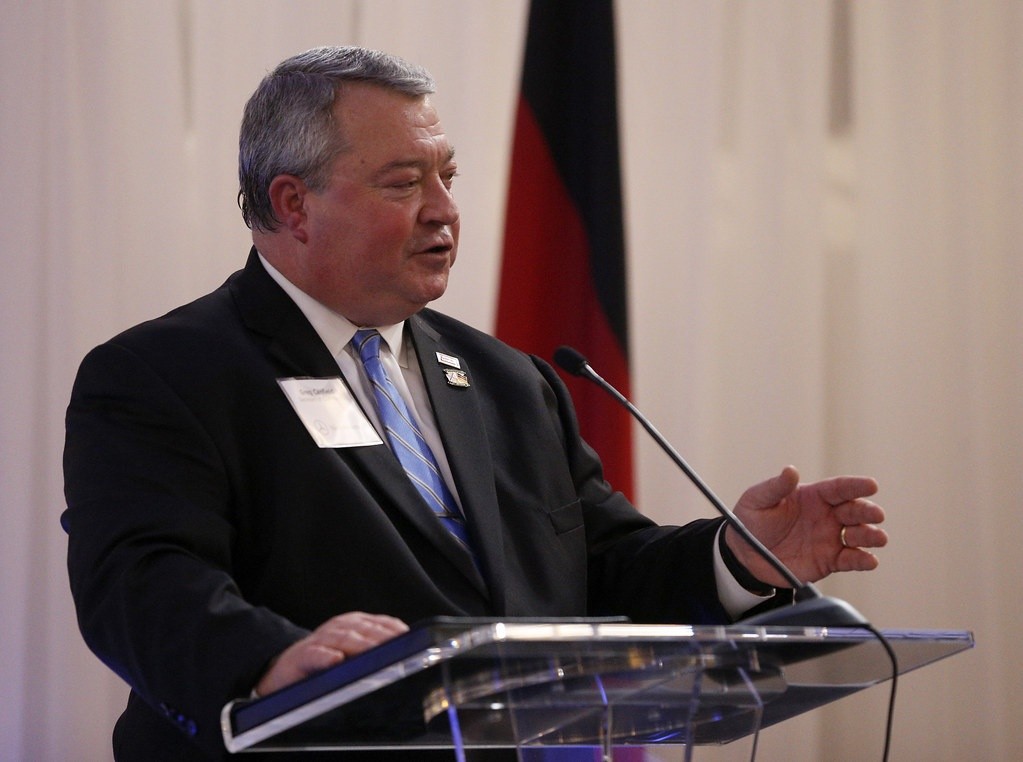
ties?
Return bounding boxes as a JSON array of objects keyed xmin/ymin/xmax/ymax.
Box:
[{"xmin": 349, "ymin": 328, "xmax": 493, "ymax": 615}]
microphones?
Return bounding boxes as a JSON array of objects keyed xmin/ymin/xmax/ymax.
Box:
[{"xmin": 552, "ymin": 346, "xmax": 871, "ymax": 626}]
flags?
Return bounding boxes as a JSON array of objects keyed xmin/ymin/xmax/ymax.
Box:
[{"xmin": 491, "ymin": 0, "xmax": 648, "ymax": 762}]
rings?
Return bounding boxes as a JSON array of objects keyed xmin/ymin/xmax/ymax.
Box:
[{"xmin": 840, "ymin": 525, "xmax": 849, "ymax": 549}]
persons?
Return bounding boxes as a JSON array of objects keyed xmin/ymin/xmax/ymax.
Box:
[{"xmin": 60, "ymin": 46, "xmax": 887, "ymax": 762}]
